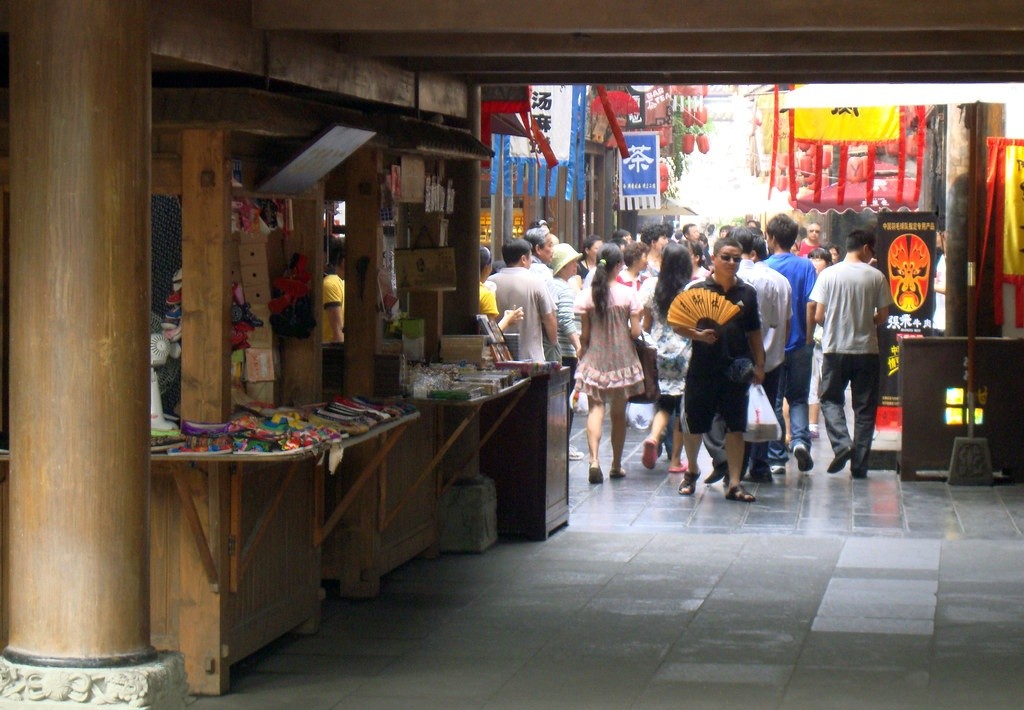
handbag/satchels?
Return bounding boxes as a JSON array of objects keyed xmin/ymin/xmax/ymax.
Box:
[
  {"xmin": 569, "ymin": 357, "xmax": 589, "ymax": 415},
  {"xmin": 626, "ymin": 328, "xmax": 661, "ymax": 403},
  {"xmin": 742, "ymin": 383, "xmax": 782, "ymax": 442}
]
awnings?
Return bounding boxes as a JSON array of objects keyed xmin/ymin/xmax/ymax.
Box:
[
  {"xmin": 794, "ymin": 158, "xmax": 921, "ymax": 214},
  {"xmin": 636, "ymin": 194, "xmax": 697, "ymax": 216}
]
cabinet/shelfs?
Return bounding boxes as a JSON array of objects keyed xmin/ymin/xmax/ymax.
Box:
[{"xmin": 481, "ymin": 365, "xmax": 573, "ymax": 541}]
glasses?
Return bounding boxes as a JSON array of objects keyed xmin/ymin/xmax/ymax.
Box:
[
  {"xmin": 809, "ymin": 229, "xmax": 820, "ymax": 233},
  {"xmin": 720, "ymin": 255, "xmax": 742, "ymax": 262},
  {"xmin": 868, "ymin": 247, "xmax": 876, "ymax": 255}
]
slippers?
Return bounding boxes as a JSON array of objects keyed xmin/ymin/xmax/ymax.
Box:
[
  {"xmin": 668, "ymin": 461, "xmax": 687, "ymax": 472},
  {"xmin": 725, "ymin": 484, "xmax": 756, "ymax": 501},
  {"xmin": 642, "ymin": 440, "xmax": 658, "ymax": 469},
  {"xmin": 678, "ymin": 468, "xmax": 701, "ymax": 494}
]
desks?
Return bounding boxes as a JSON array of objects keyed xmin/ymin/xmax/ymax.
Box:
[
  {"xmin": 376, "ymin": 376, "xmax": 536, "ymax": 530},
  {"xmin": 152, "ymin": 400, "xmax": 420, "ymax": 598}
]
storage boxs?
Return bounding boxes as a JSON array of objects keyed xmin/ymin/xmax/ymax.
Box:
[
  {"xmin": 237, "ymin": 242, "xmax": 284, "ymax": 348},
  {"xmin": 244, "ymin": 347, "xmax": 281, "ymax": 381},
  {"xmin": 245, "ymin": 382, "xmax": 281, "ymax": 406},
  {"xmin": 441, "ymin": 475, "xmax": 499, "ymax": 553}
]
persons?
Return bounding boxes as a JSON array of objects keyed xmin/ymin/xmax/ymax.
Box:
[
  {"xmin": 808, "ymin": 230, "xmax": 894, "ymax": 478},
  {"xmin": 670, "ymin": 238, "xmax": 765, "ymax": 501},
  {"xmin": 637, "ymin": 243, "xmax": 693, "ymax": 473},
  {"xmin": 322, "ymin": 259, "xmax": 346, "ymax": 344},
  {"xmin": 477, "ymin": 213, "xmax": 876, "ymax": 502},
  {"xmin": 573, "ymin": 242, "xmax": 643, "ymax": 482},
  {"xmin": 931, "ymin": 217, "xmax": 946, "ymax": 336}
]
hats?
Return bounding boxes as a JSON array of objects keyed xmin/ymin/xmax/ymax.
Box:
[{"xmin": 549, "ymin": 243, "xmax": 583, "ymax": 277}]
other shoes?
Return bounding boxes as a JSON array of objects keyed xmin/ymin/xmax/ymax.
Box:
[
  {"xmin": 704, "ymin": 462, "xmax": 727, "ymax": 482},
  {"xmin": 852, "ymin": 469, "xmax": 866, "ymax": 477},
  {"xmin": 744, "ymin": 471, "xmax": 772, "ymax": 481},
  {"xmin": 611, "ymin": 468, "xmax": 625, "ymax": 477},
  {"xmin": 589, "ymin": 462, "xmax": 603, "ymax": 483},
  {"xmin": 793, "ymin": 443, "xmax": 814, "ymax": 471},
  {"xmin": 811, "ymin": 423, "xmax": 819, "ymax": 438},
  {"xmin": 569, "ymin": 452, "xmax": 584, "ymax": 460},
  {"xmin": 770, "ymin": 465, "xmax": 785, "ymax": 473},
  {"xmin": 167, "ymin": 394, "xmax": 415, "ymax": 454},
  {"xmin": 827, "ymin": 447, "xmax": 853, "ymax": 473}
]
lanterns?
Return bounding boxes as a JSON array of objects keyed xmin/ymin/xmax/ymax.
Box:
[
  {"xmin": 775, "ymin": 140, "xmax": 831, "ymax": 192},
  {"xmin": 681, "ymin": 85, "xmax": 707, "ymax": 96},
  {"xmin": 683, "ymin": 106, "xmax": 694, "ymax": 127},
  {"xmin": 694, "ymin": 105, "xmax": 707, "ymax": 126},
  {"xmin": 696, "ymin": 133, "xmax": 710, "ymax": 154},
  {"xmin": 659, "ymin": 162, "xmax": 668, "ymax": 194},
  {"xmin": 654, "ymin": 127, "xmax": 667, "ymax": 146},
  {"xmin": 682, "ymin": 133, "xmax": 694, "ymax": 155},
  {"xmin": 788, "ymin": 197, "xmax": 797, "ymax": 208},
  {"xmin": 888, "ymin": 135, "xmax": 919, "ymax": 156}
]
